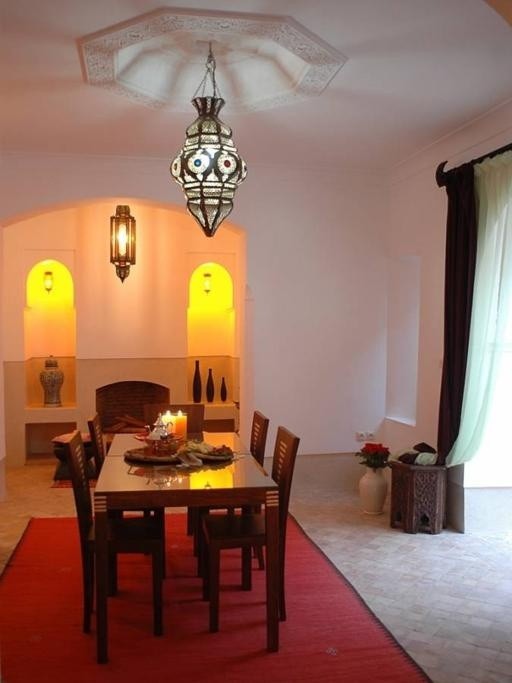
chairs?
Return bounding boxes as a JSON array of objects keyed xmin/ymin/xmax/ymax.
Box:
[
  {"xmin": 197, "ymin": 409, "xmax": 271, "ymax": 570},
  {"xmin": 142, "ymin": 403, "xmax": 207, "ymax": 433},
  {"xmin": 196, "ymin": 427, "xmax": 300, "ymax": 633},
  {"xmin": 64, "ymin": 430, "xmax": 166, "ymax": 641},
  {"xmin": 88, "ymin": 378, "xmax": 170, "ymax": 476},
  {"xmin": 84, "ymin": 411, "xmax": 165, "ymax": 600}
]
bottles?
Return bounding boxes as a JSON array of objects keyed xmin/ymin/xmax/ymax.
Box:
[
  {"xmin": 192, "ymin": 360, "xmax": 202, "ymax": 403},
  {"xmin": 206, "ymin": 368, "xmax": 215, "ymax": 403},
  {"xmin": 220, "ymin": 377, "xmax": 227, "ymax": 401}
]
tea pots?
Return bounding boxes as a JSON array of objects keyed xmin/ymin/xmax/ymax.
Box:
[{"xmin": 143, "ymin": 416, "xmax": 174, "ymax": 440}]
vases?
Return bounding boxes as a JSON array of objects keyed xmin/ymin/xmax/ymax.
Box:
[
  {"xmin": 359, "ymin": 466, "xmax": 388, "ymax": 513},
  {"xmin": 192, "ymin": 358, "xmax": 228, "ymax": 403}
]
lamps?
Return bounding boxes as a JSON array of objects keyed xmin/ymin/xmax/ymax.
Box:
[
  {"xmin": 202, "ymin": 273, "xmax": 211, "ymax": 295},
  {"xmin": 44, "ymin": 271, "xmax": 52, "ymax": 293},
  {"xmin": 108, "ymin": 204, "xmax": 137, "ymax": 283},
  {"xmin": 170, "ymin": 42, "xmax": 248, "ymax": 238}
]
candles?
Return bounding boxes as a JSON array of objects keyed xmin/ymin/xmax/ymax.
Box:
[
  {"xmin": 172, "ymin": 416, "xmax": 188, "ymax": 441},
  {"xmin": 162, "ymin": 415, "xmax": 176, "ymax": 433}
]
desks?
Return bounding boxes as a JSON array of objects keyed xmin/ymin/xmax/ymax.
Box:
[{"xmin": 52, "ymin": 431, "xmax": 98, "ymax": 481}]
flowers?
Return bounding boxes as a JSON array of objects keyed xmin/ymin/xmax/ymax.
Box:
[{"xmin": 353, "ymin": 442, "xmax": 391, "ymax": 473}]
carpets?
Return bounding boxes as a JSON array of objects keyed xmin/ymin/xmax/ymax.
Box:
[{"xmin": 0, "ymin": 507, "xmax": 432, "ymax": 682}]
